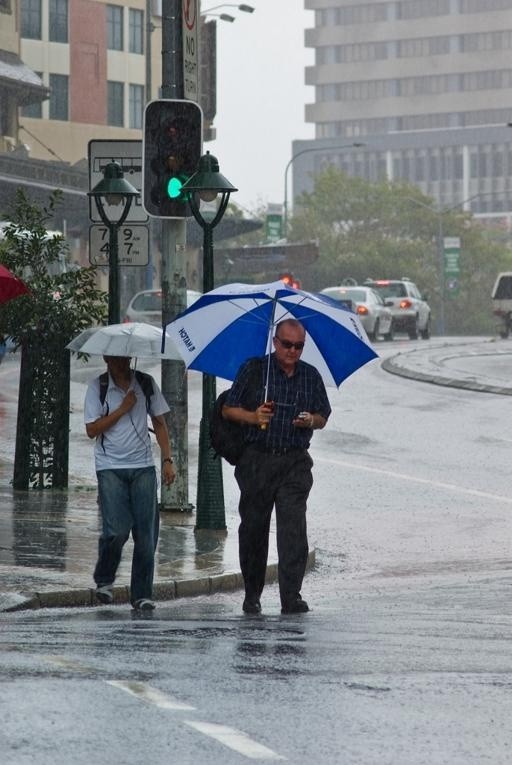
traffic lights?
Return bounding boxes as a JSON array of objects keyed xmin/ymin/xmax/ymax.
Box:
[
  {"xmin": 142, "ymin": 99, "xmax": 203, "ymax": 220},
  {"xmin": 278, "ymin": 271, "xmax": 302, "ymax": 291}
]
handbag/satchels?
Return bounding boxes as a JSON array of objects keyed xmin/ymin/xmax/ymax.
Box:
[{"xmin": 210, "ymin": 356, "xmax": 263, "ymax": 467}]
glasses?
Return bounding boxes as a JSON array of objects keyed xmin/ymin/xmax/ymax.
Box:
[{"xmin": 275, "ymin": 335, "xmax": 303, "ymax": 350}]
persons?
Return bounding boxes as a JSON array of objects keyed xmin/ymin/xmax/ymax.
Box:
[
  {"xmin": 81, "ymin": 356, "xmax": 175, "ymax": 614},
  {"xmin": 213, "ymin": 320, "xmax": 333, "ymax": 619}
]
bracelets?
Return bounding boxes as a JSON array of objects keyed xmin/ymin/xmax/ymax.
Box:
[{"xmin": 309, "ymin": 414, "xmax": 314, "ymax": 427}]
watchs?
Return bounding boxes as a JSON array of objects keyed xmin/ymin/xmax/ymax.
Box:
[{"xmin": 163, "ymin": 455, "xmax": 173, "ymax": 465}]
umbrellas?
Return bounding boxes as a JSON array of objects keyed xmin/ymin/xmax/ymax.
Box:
[
  {"xmin": 64, "ymin": 322, "xmax": 185, "ymax": 392},
  {"xmin": 1, "ymin": 264, "xmax": 29, "ymax": 308},
  {"xmin": 166, "ymin": 279, "xmax": 381, "ymax": 428}
]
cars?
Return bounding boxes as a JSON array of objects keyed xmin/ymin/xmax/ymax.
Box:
[{"xmin": 317, "ymin": 282, "xmax": 390, "ymax": 345}]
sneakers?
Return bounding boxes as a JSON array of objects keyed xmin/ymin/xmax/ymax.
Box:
[
  {"xmin": 281, "ymin": 599, "xmax": 309, "ymax": 614},
  {"xmin": 132, "ymin": 598, "xmax": 156, "ymax": 609},
  {"xmin": 243, "ymin": 597, "xmax": 262, "ymax": 613},
  {"xmin": 96, "ymin": 582, "xmax": 114, "ymax": 604}
]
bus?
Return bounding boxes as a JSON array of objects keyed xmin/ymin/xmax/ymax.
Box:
[{"xmin": 198, "ymin": 242, "xmax": 321, "ymax": 278}]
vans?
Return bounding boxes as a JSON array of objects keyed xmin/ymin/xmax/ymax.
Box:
[
  {"xmin": 488, "ymin": 271, "xmax": 511, "ymax": 342},
  {"xmin": 124, "ymin": 284, "xmax": 206, "ymax": 329}
]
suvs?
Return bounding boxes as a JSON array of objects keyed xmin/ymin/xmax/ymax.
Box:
[{"xmin": 362, "ymin": 275, "xmax": 434, "ymax": 342}]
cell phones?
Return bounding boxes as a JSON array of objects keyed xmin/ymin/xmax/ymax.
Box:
[{"xmin": 296, "ymin": 414, "xmax": 306, "ymax": 420}]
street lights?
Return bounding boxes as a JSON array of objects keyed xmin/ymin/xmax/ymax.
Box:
[
  {"xmin": 88, "ymin": 159, "xmax": 142, "ymax": 339},
  {"xmin": 398, "ymin": 190, "xmax": 510, "ymax": 340},
  {"xmin": 281, "ymin": 135, "xmax": 368, "ymax": 291},
  {"xmin": 204, "ymin": 12, "xmax": 238, "ymax": 24},
  {"xmin": 179, "ymin": 149, "xmax": 239, "ymax": 533},
  {"xmin": 200, "ymin": 1, "xmax": 257, "ymax": 13}
]
